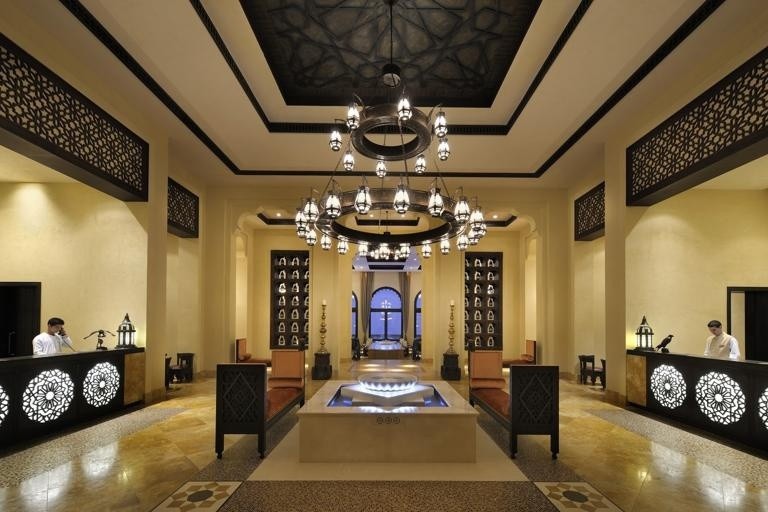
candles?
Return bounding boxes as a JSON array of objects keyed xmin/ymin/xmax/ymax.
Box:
[
  {"xmin": 308, "ymin": 297, "xmax": 334, "ymax": 382},
  {"xmin": 441, "ymin": 295, "xmax": 464, "ymax": 383}
]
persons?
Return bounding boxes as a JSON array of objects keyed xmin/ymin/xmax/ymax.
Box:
[
  {"xmin": 702, "ymin": 319, "xmax": 740, "ymax": 361},
  {"xmin": 30, "ymin": 316, "xmax": 73, "ymax": 355}
]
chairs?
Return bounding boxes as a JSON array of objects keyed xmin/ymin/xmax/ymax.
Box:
[
  {"xmin": 164, "ymin": 352, "xmax": 191, "ymax": 385},
  {"xmin": 362, "ymin": 332, "xmax": 408, "ymax": 360}
]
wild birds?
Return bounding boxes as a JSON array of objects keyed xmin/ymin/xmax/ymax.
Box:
[
  {"xmin": 655, "ymin": 334, "xmax": 674, "ymax": 351},
  {"xmin": 82, "ymin": 329, "xmax": 116, "ymax": 339}
]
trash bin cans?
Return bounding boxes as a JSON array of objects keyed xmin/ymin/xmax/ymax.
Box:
[{"xmin": 177, "ymin": 352, "xmax": 194, "ymax": 381}]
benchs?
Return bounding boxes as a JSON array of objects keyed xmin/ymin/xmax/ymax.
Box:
[
  {"xmin": 576, "ymin": 350, "xmax": 605, "ymax": 388},
  {"xmin": 466, "ymin": 339, "xmax": 560, "ymax": 463},
  {"xmin": 215, "ymin": 339, "xmax": 305, "ymax": 459}
]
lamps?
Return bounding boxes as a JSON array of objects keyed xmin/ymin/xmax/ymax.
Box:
[
  {"xmin": 292, "ymin": 0, "xmax": 489, "ymax": 259},
  {"xmin": 113, "ymin": 311, "xmax": 137, "ymax": 347},
  {"xmin": 633, "ymin": 316, "xmax": 656, "ymax": 353}
]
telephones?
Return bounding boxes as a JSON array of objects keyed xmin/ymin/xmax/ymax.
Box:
[{"xmin": 58, "ymin": 328, "xmax": 63, "ymax": 335}]
